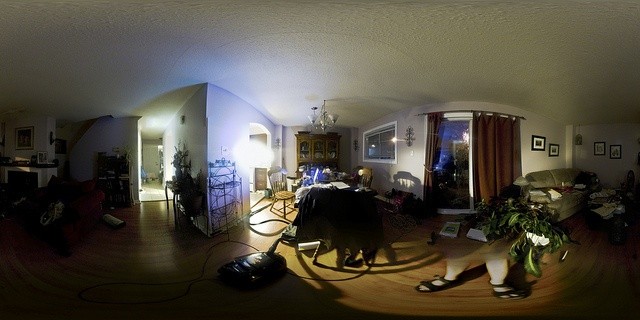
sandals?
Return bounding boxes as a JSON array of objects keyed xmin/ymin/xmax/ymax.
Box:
[
  {"xmin": 488, "ymin": 279, "xmax": 526, "ymax": 300},
  {"xmin": 415, "ymin": 275, "xmax": 460, "ymax": 292}
]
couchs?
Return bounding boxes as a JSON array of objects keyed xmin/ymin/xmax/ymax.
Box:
[
  {"xmin": 513, "ymin": 167, "xmax": 600, "ymax": 222},
  {"xmin": 22, "ymin": 181, "xmax": 105, "ymax": 241}
]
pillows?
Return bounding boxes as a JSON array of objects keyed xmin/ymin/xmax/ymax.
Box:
[{"xmin": 528, "ymin": 195, "xmax": 550, "ymax": 203}]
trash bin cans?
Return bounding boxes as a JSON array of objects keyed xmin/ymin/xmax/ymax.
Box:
[{"xmin": 264, "ymin": 189, "xmax": 272, "ymax": 198}]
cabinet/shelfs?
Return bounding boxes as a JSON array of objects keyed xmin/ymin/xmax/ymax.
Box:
[
  {"xmin": 404, "ymin": 125, "xmax": 416, "ymax": 146},
  {"xmin": 178, "ymin": 192, "xmax": 208, "ymax": 243},
  {"xmin": 294, "ymin": 131, "xmax": 342, "ymax": 178},
  {"xmin": 97, "ymin": 151, "xmax": 132, "ymax": 207},
  {"xmin": 208, "ymin": 159, "xmax": 244, "ymax": 239}
]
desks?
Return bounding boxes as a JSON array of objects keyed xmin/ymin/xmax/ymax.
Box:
[
  {"xmin": 165, "ymin": 180, "xmax": 180, "ymax": 219},
  {"xmin": 295, "ymin": 179, "xmax": 374, "ymax": 221}
]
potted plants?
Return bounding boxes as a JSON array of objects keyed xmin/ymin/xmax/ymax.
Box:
[{"xmin": 479, "ymin": 199, "xmax": 566, "ymax": 276}]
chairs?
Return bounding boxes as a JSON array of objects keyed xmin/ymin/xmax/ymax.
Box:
[{"xmin": 268, "ymin": 167, "xmax": 295, "ymax": 219}]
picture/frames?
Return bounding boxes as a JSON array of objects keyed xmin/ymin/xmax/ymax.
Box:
[
  {"xmin": 610, "ymin": 144, "xmax": 621, "ymax": 159},
  {"xmin": 594, "ymin": 141, "xmax": 606, "ymax": 156},
  {"xmin": 37, "ymin": 151, "xmax": 48, "ymax": 164},
  {"xmin": 549, "ymin": 144, "xmax": 559, "ymax": 157},
  {"xmin": 15, "ymin": 125, "xmax": 34, "ymax": 150},
  {"xmin": 531, "ymin": 135, "xmax": 546, "ymax": 151}
]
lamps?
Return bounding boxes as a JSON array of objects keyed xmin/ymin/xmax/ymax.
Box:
[
  {"xmin": 310, "ymin": 107, "xmax": 318, "ymax": 127},
  {"xmin": 312, "ymin": 100, "xmax": 338, "ymax": 133},
  {"xmin": 358, "ymin": 168, "xmax": 372, "ymax": 187}
]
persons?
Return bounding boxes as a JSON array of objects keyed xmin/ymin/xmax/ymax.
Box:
[{"xmin": 415, "ymin": 257, "xmax": 523, "ymax": 300}]
